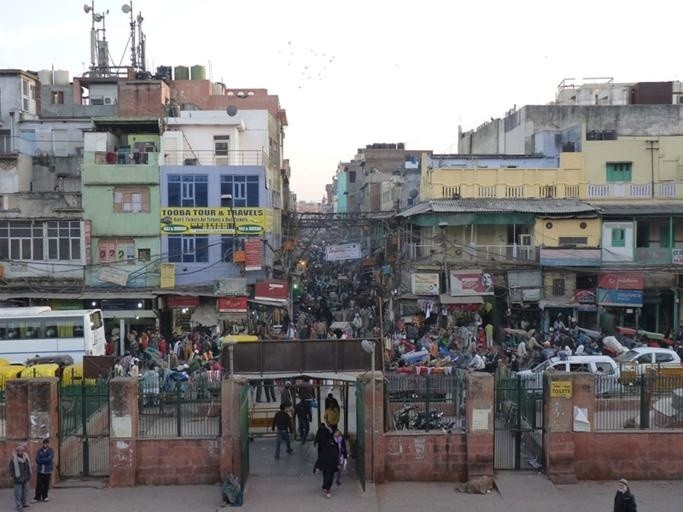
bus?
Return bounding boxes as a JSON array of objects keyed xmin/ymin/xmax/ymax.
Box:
[{"xmin": 0, "ymin": 305, "xmax": 105, "ymax": 378}]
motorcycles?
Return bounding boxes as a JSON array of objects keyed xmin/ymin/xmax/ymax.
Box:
[{"xmin": 392, "ymin": 406, "xmax": 455, "ymax": 430}]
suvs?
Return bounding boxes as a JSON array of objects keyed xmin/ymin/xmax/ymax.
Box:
[{"xmin": 508, "ymin": 351, "xmax": 623, "ymax": 402}]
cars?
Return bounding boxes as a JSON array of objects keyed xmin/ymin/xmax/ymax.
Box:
[{"xmin": 609, "ymin": 346, "xmax": 682, "ymax": 383}]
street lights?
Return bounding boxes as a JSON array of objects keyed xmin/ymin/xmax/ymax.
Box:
[
  {"xmin": 438, "ymin": 222, "xmax": 454, "ymax": 305},
  {"xmin": 359, "ymin": 339, "xmax": 377, "ymax": 484}
]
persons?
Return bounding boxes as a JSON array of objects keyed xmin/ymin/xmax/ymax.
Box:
[
  {"xmin": 406, "ymin": 310, "xmax": 683, "ymax": 369},
  {"xmin": 9, "ymin": 446, "xmax": 32, "ymax": 512},
  {"xmin": 32, "ymin": 440, "xmax": 54, "ymax": 503},
  {"xmin": 251, "ymin": 377, "xmax": 348, "ymax": 499},
  {"xmin": 221, "ymin": 318, "xmax": 266, "ymax": 339},
  {"xmin": 119, "ymin": 328, "xmax": 218, "ymax": 408},
  {"xmin": 615, "ymin": 479, "xmax": 636, "ymax": 511},
  {"xmin": 282, "ymin": 259, "xmax": 372, "ymax": 340}
]
